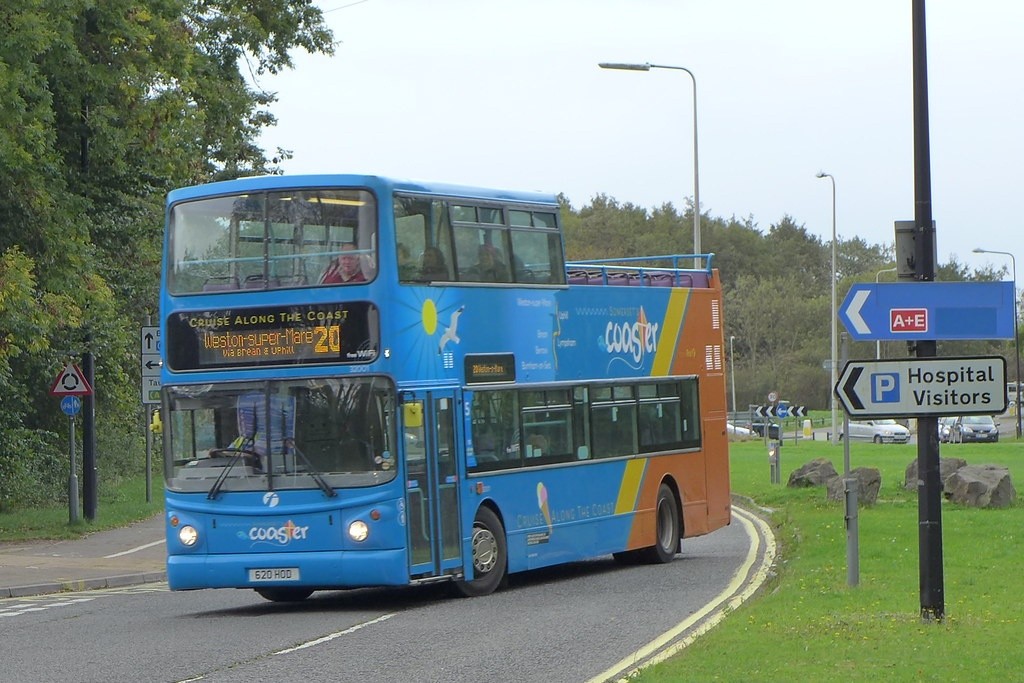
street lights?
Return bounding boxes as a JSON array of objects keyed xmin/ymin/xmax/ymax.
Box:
[
  {"xmin": 875, "ymin": 268, "xmax": 898, "ymax": 359},
  {"xmin": 597, "ymin": 60, "xmax": 702, "ymax": 269},
  {"xmin": 970, "ymin": 248, "xmax": 1022, "ymax": 439},
  {"xmin": 813, "ymin": 169, "xmax": 860, "ymax": 591},
  {"xmin": 729, "ymin": 335, "xmax": 737, "ymax": 411}
]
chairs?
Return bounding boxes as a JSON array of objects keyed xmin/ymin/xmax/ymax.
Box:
[
  {"xmin": 201, "ymin": 273, "xmax": 309, "ymax": 293},
  {"xmin": 417, "ymin": 267, "xmax": 693, "ymax": 288}
]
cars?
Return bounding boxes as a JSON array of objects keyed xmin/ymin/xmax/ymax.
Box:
[
  {"xmin": 937, "ymin": 415, "xmax": 1000, "ymax": 444},
  {"xmin": 838, "ymin": 418, "xmax": 910, "ymax": 445},
  {"xmin": 726, "ymin": 423, "xmax": 757, "ymax": 437}
]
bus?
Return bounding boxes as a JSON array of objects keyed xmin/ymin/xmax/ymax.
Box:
[{"xmin": 148, "ymin": 172, "xmax": 732, "ymax": 605}]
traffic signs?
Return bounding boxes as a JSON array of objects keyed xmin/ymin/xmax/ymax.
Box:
[
  {"xmin": 832, "ymin": 355, "xmax": 1010, "ymax": 420},
  {"xmin": 140, "ymin": 325, "xmax": 161, "ymax": 354},
  {"xmin": 837, "ymin": 280, "xmax": 1016, "ymax": 343},
  {"xmin": 141, "ymin": 354, "xmax": 162, "ymax": 376}
]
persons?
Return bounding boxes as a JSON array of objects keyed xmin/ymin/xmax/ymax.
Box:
[
  {"xmin": 207, "ymin": 412, "xmax": 267, "ymax": 458},
  {"xmin": 510, "ymin": 417, "xmax": 551, "ymax": 458},
  {"xmin": 397, "ymin": 243, "xmax": 528, "ymax": 283},
  {"xmin": 321, "ymin": 243, "xmax": 368, "ymax": 285}
]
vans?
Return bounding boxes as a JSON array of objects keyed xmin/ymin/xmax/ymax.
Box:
[{"xmin": 1006, "ymin": 383, "xmax": 1024, "ymax": 408}]
